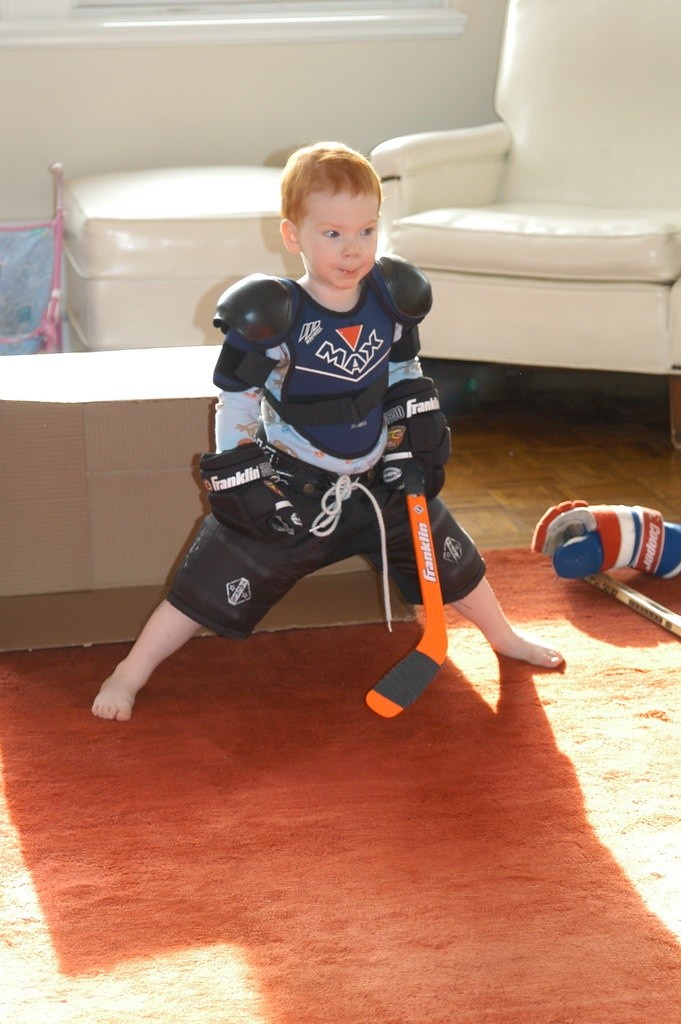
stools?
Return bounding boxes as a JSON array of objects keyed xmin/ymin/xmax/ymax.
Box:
[{"xmin": 57, "ymin": 164, "xmax": 318, "ymax": 356}]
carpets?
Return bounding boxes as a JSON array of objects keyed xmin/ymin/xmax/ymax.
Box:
[{"xmin": 0, "ymin": 544, "xmax": 680, "ymax": 1024}]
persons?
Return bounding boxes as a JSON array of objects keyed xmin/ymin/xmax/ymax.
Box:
[{"xmin": 91, "ymin": 141, "xmax": 562, "ymax": 722}]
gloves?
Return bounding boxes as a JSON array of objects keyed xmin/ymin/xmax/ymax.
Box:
[
  {"xmin": 359, "ymin": 376, "xmax": 451, "ymax": 524},
  {"xmin": 200, "ymin": 442, "xmax": 304, "ymax": 542}
]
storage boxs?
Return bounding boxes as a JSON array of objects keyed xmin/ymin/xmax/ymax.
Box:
[{"xmin": 0, "ymin": 345, "xmax": 416, "ymax": 654}]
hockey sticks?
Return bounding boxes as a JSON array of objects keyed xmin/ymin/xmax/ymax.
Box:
[
  {"xmin": 579, "ymin": 571, "xmax": 681, "ymax": 638},
  {"xmin": 365, "ymin": 466, "xmax": 448, "ymax": 719}
]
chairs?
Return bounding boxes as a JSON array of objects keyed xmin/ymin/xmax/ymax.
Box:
[{"xmin": 366, "ymin": 0, "xmax": 681, "ymax": 449}]
